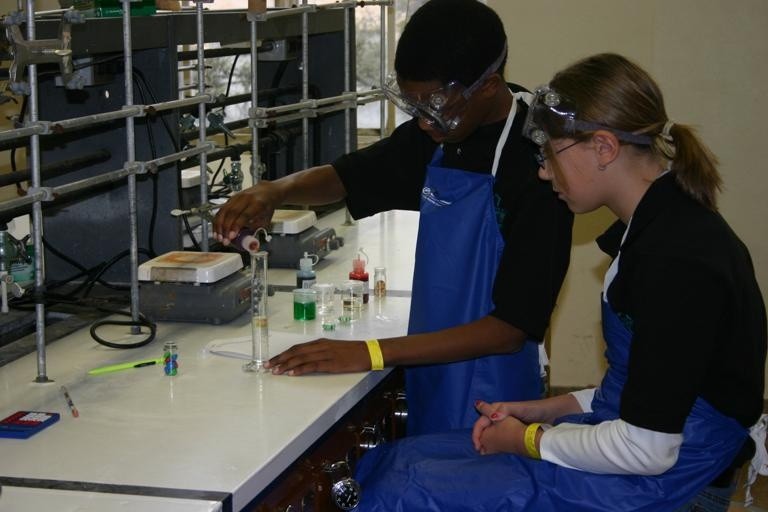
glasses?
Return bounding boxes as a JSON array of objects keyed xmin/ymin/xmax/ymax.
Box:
[
  {"xmin": 534, "ymin": 139, "xmax": 588, "ymax": 170},
  {"xmin": 523, "ymin": 86, "xmax": 651, "ymax": 152},
  {"xmin": 383, "ymin": 71, "xmax": 487, "ymax": 134}
]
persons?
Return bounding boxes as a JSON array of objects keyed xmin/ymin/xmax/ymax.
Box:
[
  {"xmin": 358, "ymin": 52, "xmax": 768, "ymax": 511},
  {"xmin": 213, "ymin": 2, "xmax": 575, "ymax": 431}
]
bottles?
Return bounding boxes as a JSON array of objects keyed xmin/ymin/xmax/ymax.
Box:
[
  {"xmin": 161, "ymin": 341, "xmax": 179, "ymax": 375},
  {"xmin": 230, "ymin": 227, "xmax": 386, "ymax": 374}
]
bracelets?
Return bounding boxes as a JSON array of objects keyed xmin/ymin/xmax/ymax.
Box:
[
  {"xmin": 365, "ymin": 339, "xmax": 384, "ymax": 371},
  {"xmin": 525, "ymin": 423, "xmax": 553, "ymax": 458}
]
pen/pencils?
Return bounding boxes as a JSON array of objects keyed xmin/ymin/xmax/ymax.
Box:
[
  {"xmin": 209, "ymin": 349, "xmax": 251, "ymax": 360},
  {"xmin": 60, "ymin": 386, "xmax": 79, "ymax": 418},
  {"xmin": 90, "ymin": 358, "xmax": 163, "ymax": 375}
]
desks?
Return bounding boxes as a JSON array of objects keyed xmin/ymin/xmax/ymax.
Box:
[{"xmin": 0, "ymin": 208, "xmax": 421, "ymax": 511}]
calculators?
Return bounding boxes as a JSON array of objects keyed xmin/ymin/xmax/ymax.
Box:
[{"xmin": 0, "ymin": 411, "xmax": 58, "ymax": 439}]
